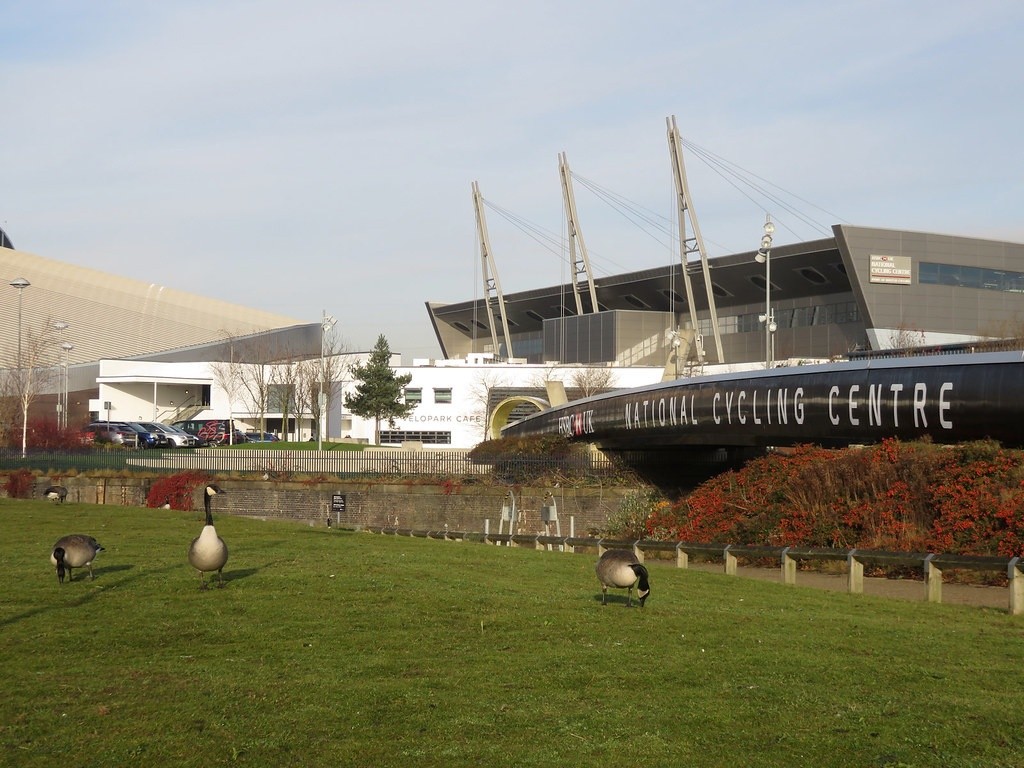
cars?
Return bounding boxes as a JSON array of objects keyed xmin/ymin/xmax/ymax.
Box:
[
  {"xmin": 131, "ymin": 421, "xmax": 195, "ymax": 448},
  {"xmin": 170, "ymin": 427, "xmax": 205, "ymax": 449},
  {"xmin": 93, "ymin": 420, "xmax": 168, "ymax": 449}
]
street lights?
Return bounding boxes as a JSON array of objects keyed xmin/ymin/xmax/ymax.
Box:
[
  {"xmin": 8, "ymin": 274, "xmax": 32, "ymax": 380},
  {"xmin": 216, "ymin": 326, "xmax": 235, "ymax": 446},
  {"xmin": 669, "ymin": 326, "xmax": 680, "ymax": 379},
  {"xmin": 755, "ymin": 213, "xmax": 774, "ymax": 367},
  {"xmin": 759, "ymin": 308, "xmax": 776, "ymax": 369},
  {"xmin": 52, "ymin": 319, "xmax": 74, "ymax": 430},
  {"xmin": 697, "ymin": 334, "xmax": 705, "ymax": 375},
  {"xmin": 317, "ymin": 313, "xmax": 340, "ymax": 450}
]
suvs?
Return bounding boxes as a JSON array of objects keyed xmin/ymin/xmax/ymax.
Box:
[{"xmin": 87, "ymin": 424, "xmax": 139, "ymax": 446}]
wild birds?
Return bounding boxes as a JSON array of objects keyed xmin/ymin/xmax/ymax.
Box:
[
  {"xmin": 595, "ymin": 549, "xmax": 651, "ymax": 609},
  {"xmin": 41, "ymin": 485, "xmax": 70, "ymax": 505},
  {"xmin": 156, "ymin": 494, "xmax": 171, "ymax": 510},
  {"xmin": 48, "ymin": 533, "xmax": 108, "ymax": 586},
  {"xmin": 188, "ymin": 483, "xmax": 229, "ymax": 592}
]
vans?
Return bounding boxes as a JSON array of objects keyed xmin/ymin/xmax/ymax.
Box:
[{"xmin": 171, "ymin": 419, "xmax": 236, "ymax": 449}]
